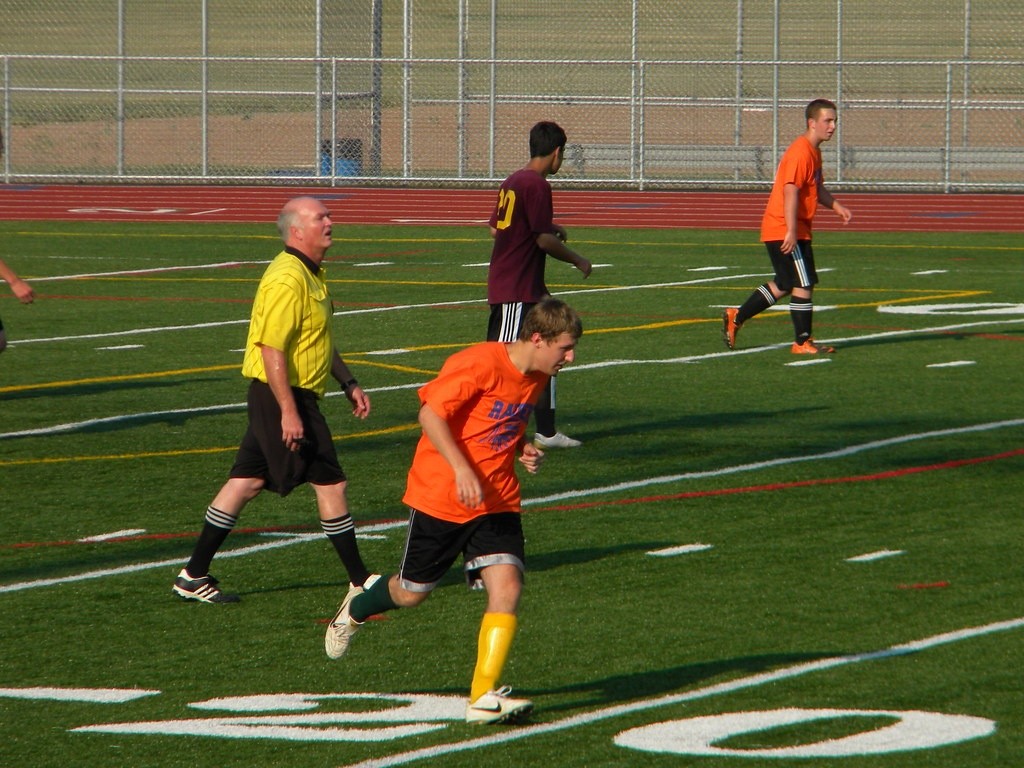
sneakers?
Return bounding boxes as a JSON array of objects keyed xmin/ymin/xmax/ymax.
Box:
[
  {"xmin": 324, "ymin": 585, "xmax": 367, "ymax": 660},
  {"xmin": 349, "ymin": 574, "xmax": 384, "ymax": 594},
  {"xmin": 789, "ymin": 334, "xmax": 836, "ymax": 355},
  {"xmin": 170, "ymin": 564, "xmax": 241, "ymax": 604},
  {"xmin": 465, "ymin": 684, "xmax": 535, "ymax": 726},
  {"xmin": 534, "ymin": 430, "xmax": 584, "ymax": 450},
  {"xmin": 721, "ymin": 307, "xmax": 746, "ymax": 351}
]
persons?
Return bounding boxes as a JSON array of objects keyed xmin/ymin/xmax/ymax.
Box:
[
  {"xmin": 171, "ymin": 197, "xmax": 385, "ymax": 603},
  {"xmin": 487, "ymin": 121, "xmax": 592, "ymax": 449},
  {"xmin": 0, "ymin": 259, "xmax": 37, "ymax": 354},
  {"xmin": 723, "ymin": 99, "xmax": 852, "ymax": 355},
  {"xmin": 324, "ymin": 297, "xmax": 582, "ymax": 724}
]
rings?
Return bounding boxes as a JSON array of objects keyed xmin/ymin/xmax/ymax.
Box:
[{"xmin": 293, "ymin": 438, "xmax": 305, "ymax": 443}]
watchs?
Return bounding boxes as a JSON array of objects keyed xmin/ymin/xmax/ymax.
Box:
[{"xmin": 340, "ymin": 379, "xmax": 358, "ymax": 391}]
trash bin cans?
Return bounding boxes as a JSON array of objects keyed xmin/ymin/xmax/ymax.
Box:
[{"xmin": 318, "ymin": 137, "xmax": 363, "ymax": 178}]
